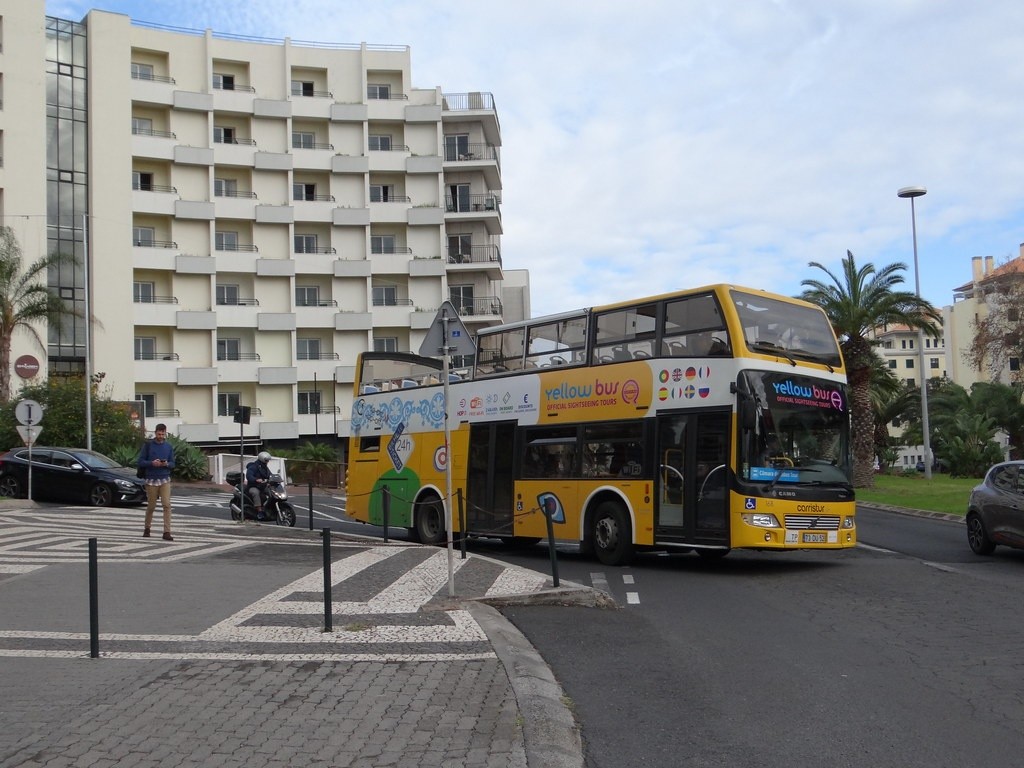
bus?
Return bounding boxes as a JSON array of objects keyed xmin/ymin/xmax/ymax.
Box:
[{"xmin": 343, "ymin": 282, "xmax": 856, "ymax": 567}]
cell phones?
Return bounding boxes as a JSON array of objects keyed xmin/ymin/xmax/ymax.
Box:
[{"xmin": 159, "ymin": 460, "xmax": 166, "ymax": 462}]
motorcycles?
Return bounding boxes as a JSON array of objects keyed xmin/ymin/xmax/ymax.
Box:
[{"xmin": 225, "ymin": 451, "xmax": 297, "ymax": 528}]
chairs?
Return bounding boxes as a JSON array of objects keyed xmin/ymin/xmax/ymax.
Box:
[
  {"xmin": 474, "ymin": 152, "xmax": 482, "ymax": 160},
  {"xmin": 460, "ymin": 154, "xmax": 467, "ymax": 161},
  {"xmin": 363, "ymin": 336, "xmax": 730, "ymax": 394},
  {"xmin": 462, "ymin": 255, "xmax": 471, "ymax": 264},
  {"xmin": 458, "ymin": 307, "xmax": 468, "ymax": 316}
]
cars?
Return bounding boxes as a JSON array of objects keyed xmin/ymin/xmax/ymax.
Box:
[
  {"xmin": 966, "ymin": 459, "xmax": 1024, "ymax": 556},
  {"xmin": 0, "ymin": 445, "xmax": 160, "ymax": 509}
]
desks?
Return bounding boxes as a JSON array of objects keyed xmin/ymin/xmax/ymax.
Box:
[
  {"xmin": 472, "ymin": 204, "xmax": 482, "ymax": 212},
  {"xmin": 465, "ymin": 153, "xmax": 474, "ymax": 160}
]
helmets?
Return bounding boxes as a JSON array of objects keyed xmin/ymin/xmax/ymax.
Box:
[{"xmin": 258, "ymin": 451, "xmax": 271, "ymax": 463}]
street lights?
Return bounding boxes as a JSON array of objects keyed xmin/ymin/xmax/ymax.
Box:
[{"xmin": 896, "ymin": 183, "xmax": 931, "ymax": 477}]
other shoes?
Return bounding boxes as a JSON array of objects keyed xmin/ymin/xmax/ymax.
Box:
[
  {"xmin": 143, "ymin": 529, "xmax": 150, "ymax": 537},
  {"xmin": 162, "ymin": 532, "xmax": 173, "ymax": 541},
  {"xmin": 256, "ymin": 511, "xmax": 264, "ymax": 519}
]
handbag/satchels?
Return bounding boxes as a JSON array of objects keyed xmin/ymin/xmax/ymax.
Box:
[{"xmin": 137, "ymin": 467, "xmax": 145, "ymax": 479}]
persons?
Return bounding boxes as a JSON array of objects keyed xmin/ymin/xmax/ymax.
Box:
[
  {"xmin": 246, "ymin": 452, "xmax": 273, "ymax": 519},
  {"xmin": 138, "ymin": 424, "xmax": 175, "ymax": 540},
  {"xmin": 752, "ymin": 435, "xmax": 804, "ymax": 468}
]
